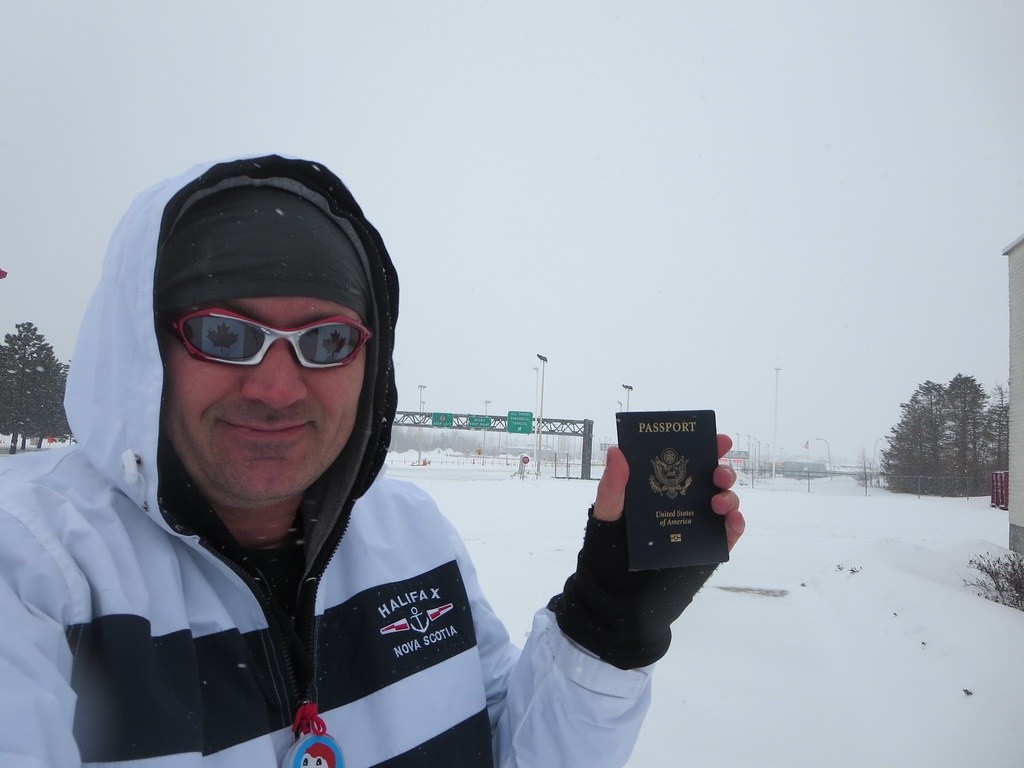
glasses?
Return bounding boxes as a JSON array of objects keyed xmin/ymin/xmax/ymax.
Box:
[{"xmin": 162, "ymin": 305, "xmax": 372, "ymax": 367}]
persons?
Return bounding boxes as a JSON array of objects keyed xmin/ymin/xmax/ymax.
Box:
[{"xmin": 0, "ymin": 155, "xmax": 746, "ymax": 768}]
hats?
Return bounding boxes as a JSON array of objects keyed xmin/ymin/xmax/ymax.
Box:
[{"xmin": 156, "ymin": 186, "xmax": 373, "ymax": 323}]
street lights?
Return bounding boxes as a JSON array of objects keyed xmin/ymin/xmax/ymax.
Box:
[
  {"xmin": 621, "ymin": 384, "xmax": 634, "ymax": 410},
  {"xmin": 481, "ymin": 399, "xmax": 490, "ymax": 458},
  {"xmin": 773, "ymin": 366, "xmax": 782, "ymax": 475},
  {"xmin": 735, "ymin": 433, "xmax": 760, "ymax": 472},
  {"xmin": 816, "ymin": 438, "xmax": 832, "ymax": 479},
  {"xmin": 537, "ymin": 353, "xmax": 547, "ymax": 480},
  {"xmin": 871, "ymin": 439, "xmax": 880, "ymax": 486},
  {"xmin": 419, "ymin": 385, "xmax": 427, "ymax": 466}
]
